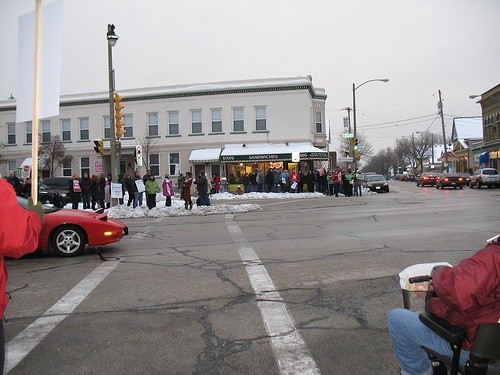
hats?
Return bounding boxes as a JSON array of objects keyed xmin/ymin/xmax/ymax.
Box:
[{"xmin": 146, "ymin": 169, "xmax": 151, "ymax": 171}]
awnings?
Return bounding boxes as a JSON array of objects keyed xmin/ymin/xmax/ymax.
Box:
[
  {"xmin": 19, "ymin": 156, "xmax": 50, "ymax": 172},
  {"xmin": 221, "ymin": 143, "xmax": 329, "ymax": 163},
  {"xmin": 188, "ymin": 149, "xmax": 221, "ymax": 162}
]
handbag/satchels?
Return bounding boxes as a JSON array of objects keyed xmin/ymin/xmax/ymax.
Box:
[
  {"xmin": 282, "ymin": 177, "xmax": 286, "ymax": 183},
  {"xmin": 73, "ymin": 180, "xmax": 81, "ymax": 192}
]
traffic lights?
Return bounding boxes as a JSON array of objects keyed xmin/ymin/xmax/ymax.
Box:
[
  {"xmin": 354, "ymin": 138, "xmax": 358, "ymax": 150},
  {"xmin": 115, "ymin": 95, "xmax": 126, "ymax": 138},
  {"xmin": 345, "ymin": 151, "xmax": 349, "ymax": 155},
  {"xmin": 93, "ymin": 138, "xmax": 103, "ymax": 154}
]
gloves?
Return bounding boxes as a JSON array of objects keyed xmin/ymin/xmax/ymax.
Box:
[{"xmin": 430, "ymin": 265, "xmax": 449, "ymax": 277}]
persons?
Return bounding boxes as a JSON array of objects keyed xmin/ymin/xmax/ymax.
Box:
[
  {"xmin": 387, "ymin": 235, "xmax": 499, "ymax": 374},
  {"xmin": 2, "ymin": 169, "xmax": 32, "ymax": 197},
  {"xmin": 241, "ymin": 165, "xmax": 365, "ymax": 197},
  {"xmin": 0, "ymin": 175, "xmax": 46, "ymax": 372},
  {"xmin": 194, "ymin": 170, "xmax": 213, "ymax": 206},
  {"xmin": 180, "ymin": 172, "xmax": 193, "ymax": 210},
  {"xmin": 67, "ymin": 169, "xmax": 158, "ymax": 213},
  {"xmin": 161, "ymin": 174, "xmax": 175, "ymax": 207},
  {"xmin": 213, "ymin": 174, "xmax": 220, "ymax": 193}
]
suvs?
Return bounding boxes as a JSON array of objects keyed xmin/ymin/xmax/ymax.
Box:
[{"xmin": 468, "ymin": 167, "xmax": 500, "ymax": 189}]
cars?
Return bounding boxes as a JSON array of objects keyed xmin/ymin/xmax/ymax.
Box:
[
  {"xmin": 360, "ymin": 170, "xmax": 443, "ymax": 193},
  {"xmin": 458, "ymin": 173, "xmax": 471, "ymax": 186},
  {"xmin": 436, "ymin": 173, "xmax": 463, "ymax": 190},
  {"xmin": 15, "ymin": 195, "xmax": 129, "ymax": 258}
]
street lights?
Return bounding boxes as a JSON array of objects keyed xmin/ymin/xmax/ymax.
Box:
[
  {"xmin": 416, "ymin": 131, "xmax": 434, "ymax": 165},
  {"xmin": 106, "ymin": 23, "xmax": 120, "ymax": 183},
  {"xmin": 352, "ymin": 78, "xmax": 390, "ymax": 181}
]
